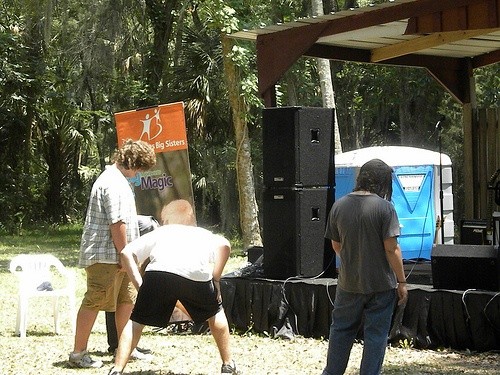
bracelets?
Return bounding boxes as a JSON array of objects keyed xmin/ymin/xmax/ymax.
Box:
[{"xmin": 398, "ymin": 281, "xmax": 407, "ymax": 284}]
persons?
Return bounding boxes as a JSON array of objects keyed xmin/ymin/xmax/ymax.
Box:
[
  {"xmin": 323, "ymin": 158, "xmax": 409, "ymax": 375},
  {"xmin": 68, "ymin": 138, "xmax": 155, "ymax": 368},
  {"xmin": 105, "ymin": 201, "xmax": 238, "ymax": 375}
]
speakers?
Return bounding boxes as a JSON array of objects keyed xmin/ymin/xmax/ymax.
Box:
[
  {"xmin": 430, "ymin": 244, "xmax": 500, "ymax": 289},
  {"xmin": 263, "ymin": 106, "xmax": 336, "ymax": 280},
  {"xmin": 460, "ymin": 218, "xmax": 495, "ymax": 245}
]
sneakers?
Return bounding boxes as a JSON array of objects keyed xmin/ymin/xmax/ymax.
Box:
[
  {"xmin": 221, "ymin": 360, "xmax": 243, "ymax": 375},
  {"xmin": 108, "ymin": 366, "xmax": 124, "ymax": 375},
  {"xmin": 112, "ymin": 348, "xmax": 145, "ymax": 361},
  {"xmin": 67, "ymin": 349, "xmax": 104, "ymax": 368}
]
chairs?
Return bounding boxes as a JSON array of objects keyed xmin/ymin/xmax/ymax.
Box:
[{"xmin": 10, "ymin": 254, "xmax": 77, "ymax": 337}]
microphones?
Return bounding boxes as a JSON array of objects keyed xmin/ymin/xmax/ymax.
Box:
[{"xmin": 435, "ymin": 115, "xmax": 445, "ymax": 128}]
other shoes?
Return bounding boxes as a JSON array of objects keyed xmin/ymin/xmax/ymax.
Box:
[{"xmin": 108, "ymin": 344, "xmax": 152, "ymax": 355}]
